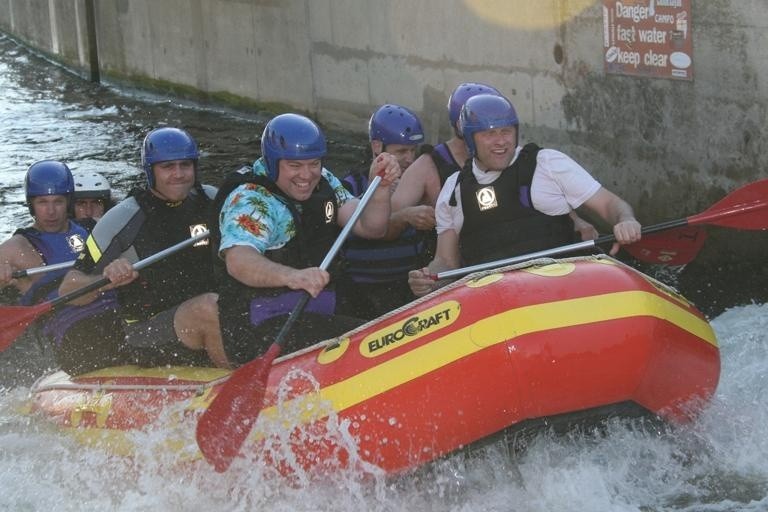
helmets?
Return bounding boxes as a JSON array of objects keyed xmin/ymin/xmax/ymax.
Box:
[
  {"xmin": 72, "ymin": 173, "xmax": 110, "ymax": 199},
  {"xmin": 26, "ymin": 159, "xmax": 74, "ymax": 216},
  {"xmin": 450, "ymin": 82, "xmax": 500, "ymax": 122},
  {"xmin": 261, "ymin": 114, "xmax": 327, "ymax": 184},
  {"xmin": 144, "ymin": 128, "xmax": 198, "ymax": 188},
  {"xmin": 369, "ymin": 104, "xmax": 424, "ymax": 145},
  {"xmin": 460, "ymin": 95, "xmax": 519, "ymax": 157}
]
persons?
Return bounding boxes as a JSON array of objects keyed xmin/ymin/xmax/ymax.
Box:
[
  {"xmin": 1, "ymin": 161, "xmax": 129, "ymax": 378},
  {"xmin": 381, "ymin": 82, "xmax": 621, "ymax": 290},
  {"xmin": 407, "ymin": 95, "xmax": 643, "ymax": 300},
  {"xmin": 323, "ymin": 103, "xmax": 425, "ymax": 323},
  {"xmin": 70, "ymin": 172, "xmax": 117, "ymax": 222},
  {"xmin": 207, "ymin": 112, "xmax": 403, "ymax": 367},
  {"xmin": 57, "ymin": 127, "xmax": 231, "ymax": 368}
]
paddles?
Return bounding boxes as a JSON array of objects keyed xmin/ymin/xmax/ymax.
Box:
[
  {"xmin": 574, "ymin": 226, "xmax": 708, "ymax": 264},
  {"xmin": 0, "ymin": 228, "xmax": 210, "ymax": 354},
  {"xmin": 423, "ymin": 178, "xmax": 767, "ymax": 280},
  {"xmin": 196, "ymin": 159, "xmax": 385, "ymax": 473}
]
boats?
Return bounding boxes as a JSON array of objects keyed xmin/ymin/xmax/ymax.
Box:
[{"xmin": 23, "ymin": 254, "xmax": 721, "ymax": 504}]
